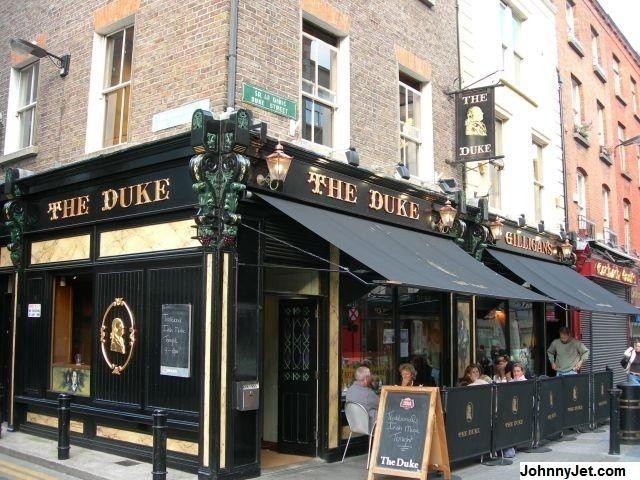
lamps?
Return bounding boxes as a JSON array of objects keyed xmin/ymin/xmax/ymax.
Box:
[
  {"xmin": 329, "ymin": 146, "xmax": 410, "ymax": 179},
  {"xmin": 256, "ymin": 136, "xmax": 295, "ymax": 190},
  {"xmin": 431, "ymin": 196, "xmax": 457, "ymax": 233},
  {"xmin": 549, "ymin": 238, "xmax": 573, "ymax": 259},
  {"xmin": 10, "ymin": 36, "xmax": 70, "ymax": 80}
]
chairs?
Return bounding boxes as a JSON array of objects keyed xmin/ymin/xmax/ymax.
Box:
[{"xmin": 341, "ymin": 401, "xmax": 380, "ymax": 469}]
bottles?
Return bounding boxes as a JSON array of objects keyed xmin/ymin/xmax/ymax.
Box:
[{"xmin": 75, "ymin": 354, "xmax": 82, "ymax": 366}]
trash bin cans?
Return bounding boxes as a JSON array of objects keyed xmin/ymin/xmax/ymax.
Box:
[{"xmin": 612, "ymin": 381, "xmax": 640, "ymax": 444}]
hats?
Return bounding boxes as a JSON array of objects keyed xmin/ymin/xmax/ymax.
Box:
[{"xmin": 494, "ymin": 357, "xmax": 506, "ymax": 363}]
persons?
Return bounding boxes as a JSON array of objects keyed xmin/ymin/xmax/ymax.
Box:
[
  {"xmin": 624, "ymin": 341, "xmax": 640, "ymax": 384},
  {"xmin": 426, "ymin": 328, "xmax": 441, "ymax": 387},
  {"xmin": 547, "ymin": 327, "xmax": 589, "ymax": 376},
  {"xmin": 399, "ymin": 363, "xmax": 418, "ymax": 386},
  {"xmin": 346, "ymin": 367, "xmax": 380, "ymax": 435},
  {"xmin": 457, "ymin": 310, "xmax": 468, "ymax": 369},
  {"xmin": 477, "ymin": 343, "xmax": 529, "ymax": 366},
  {"xmin": 349, "ymin": 359, "xmax": 379, "ymax": 390},
  {"xmin": 458, "ymin": 356, "xmax": 533, "ymax": 386}
]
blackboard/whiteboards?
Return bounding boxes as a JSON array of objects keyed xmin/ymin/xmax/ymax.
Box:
[
  {"xmin": 160, "ymin": 303, "xmax": 192, "ymax": 378},
  {"xmin": 369, "ymin": 386, "xmax": 439, "ymax": 478}
]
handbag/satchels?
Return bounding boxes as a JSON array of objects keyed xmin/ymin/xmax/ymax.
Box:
[{"xmin": 621, "ymin": 351, "xmax": 632, "ymax": 368}]
